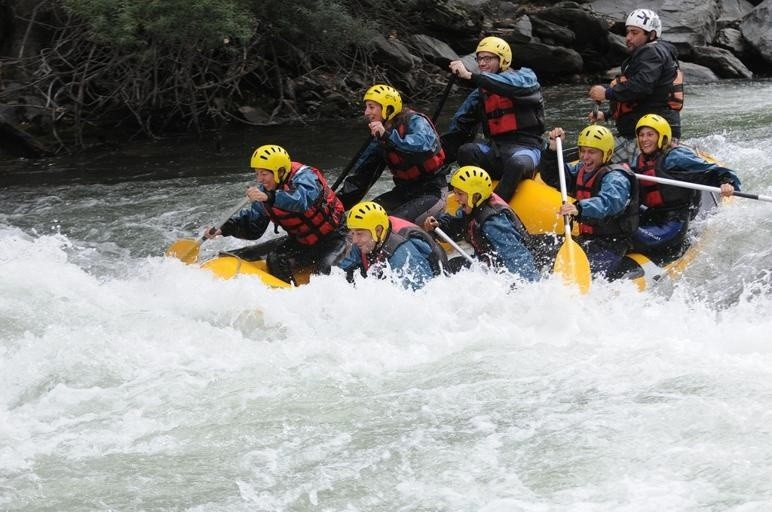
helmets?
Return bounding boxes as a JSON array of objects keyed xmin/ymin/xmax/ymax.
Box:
[
  {"xmin": 363, "ymin": 85, "xmax": 402, "ymax": 120},
  {"xmin": 450, "ymin": 166, "xmax": 492, "ymax": 208},
  {"xmin": 347, "ymin": 202, "xmax": 389, "ymax": 242},
  {"xmin": 476, "ymin": 37, "xmax": 512, "ymax": 71},
  {"xmin": 635, "ymin": 113, "xmax": 672, "ymax": 149},
  {"xmin": 251, "ymin": 145, "xmax": 292, "ymax": 184},
  {"xmin": 625, "ymin": 9, "xmax": 662, "ymax": 38},
  {"xmin": 577, "ymin": 125, "xmax": 614, "ymax": 164}
]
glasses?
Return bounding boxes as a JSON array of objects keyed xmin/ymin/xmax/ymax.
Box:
[{"xmin": 475, "ymin": 56, "xmax": 497, "ymax": 63}]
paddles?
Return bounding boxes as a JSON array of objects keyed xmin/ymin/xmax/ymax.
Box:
[
  {"xmin": 166, "ymin": 196, "xmax": 251, "ymax": 265},
  {"xmin": 553, "ymin": 136, "xmax": 591, "ymax": 291}
]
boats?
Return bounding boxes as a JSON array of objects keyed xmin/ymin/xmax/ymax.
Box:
[{"xmin": 200, "ymin": 145, "xmax": 739, "ymax": 294}]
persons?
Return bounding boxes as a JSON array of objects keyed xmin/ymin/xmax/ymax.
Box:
[
  {"xmin": 425, "ymin": 165, "xmax": 555, "ymax": 283},
  {"xmin": 629, "ymin": 113, "xmax": 743, "ymax": 263},
  {"xmin": 204, "ymin": 145, "xmax": 350, "ymax": 287},
  {"xmin": 541, "ymin": 125, "xmax": 640, "ymax": 282},
  {"xmin": 336, "ymin": 202, "xmax": 450, "ymax": 293},
  {"xmin": 335, "ymin": 84, "xmax": 451, "ymax": 229},
  {"xmin": 440, "ymin": 36, "xmax": 548, "ymax": 204},
  {"xmin": 586, "ymin": 9, "xmax": 685, "ymax": 166}
]
